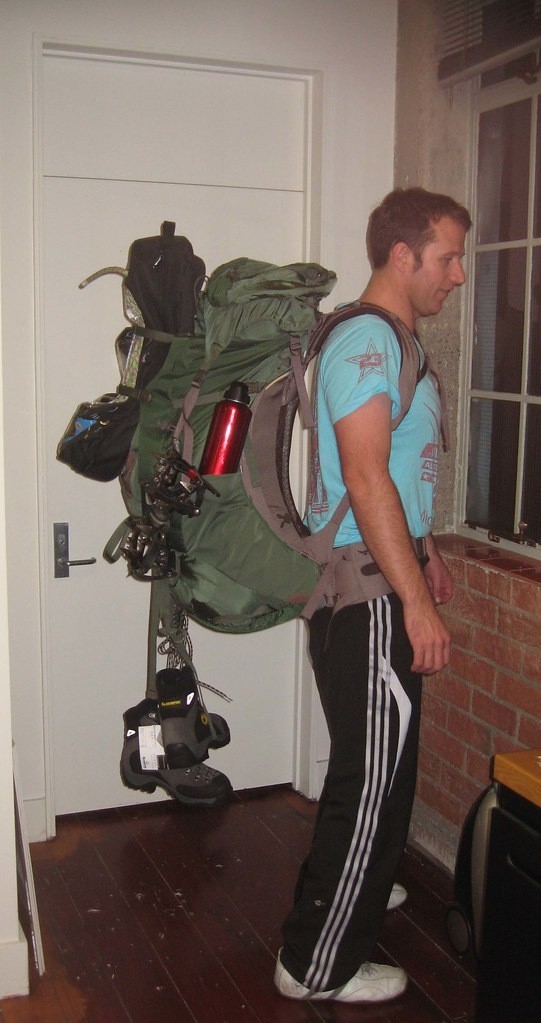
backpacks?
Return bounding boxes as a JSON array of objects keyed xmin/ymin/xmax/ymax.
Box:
[{"xmin": 118, "ymin": 254, "xmax": 417, "ymax": 635}]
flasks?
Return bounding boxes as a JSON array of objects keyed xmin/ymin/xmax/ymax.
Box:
[{"xmin": 199, "ymin": 379, "xmax": 257, "ymax": 486}]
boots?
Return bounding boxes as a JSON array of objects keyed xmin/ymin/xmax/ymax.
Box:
[
  {"xmin": 156, "ymin": 666, "xmax": 230, "ymax": 771},
  {"xmin": 120, "ymin": 699, "xmax": 232, "ymax": 807}
]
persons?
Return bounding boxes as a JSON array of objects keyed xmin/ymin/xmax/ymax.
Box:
[{"xmin": 274, "ymin": 187, "xmax": 473, "ymax": 1006}]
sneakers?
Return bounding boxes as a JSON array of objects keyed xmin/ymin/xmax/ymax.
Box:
[
  {"xmin": 387, "ymin": 884, "xmax": 408, "ymax": 910},
  {"xmin": 274, "ymin": 946, "xmax": 407, "ymax": 1002}
]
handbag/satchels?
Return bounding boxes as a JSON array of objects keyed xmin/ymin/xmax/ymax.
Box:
[{"xmin": 56, "ymin": 392, "xmax": 140, "ymax": 482}]
